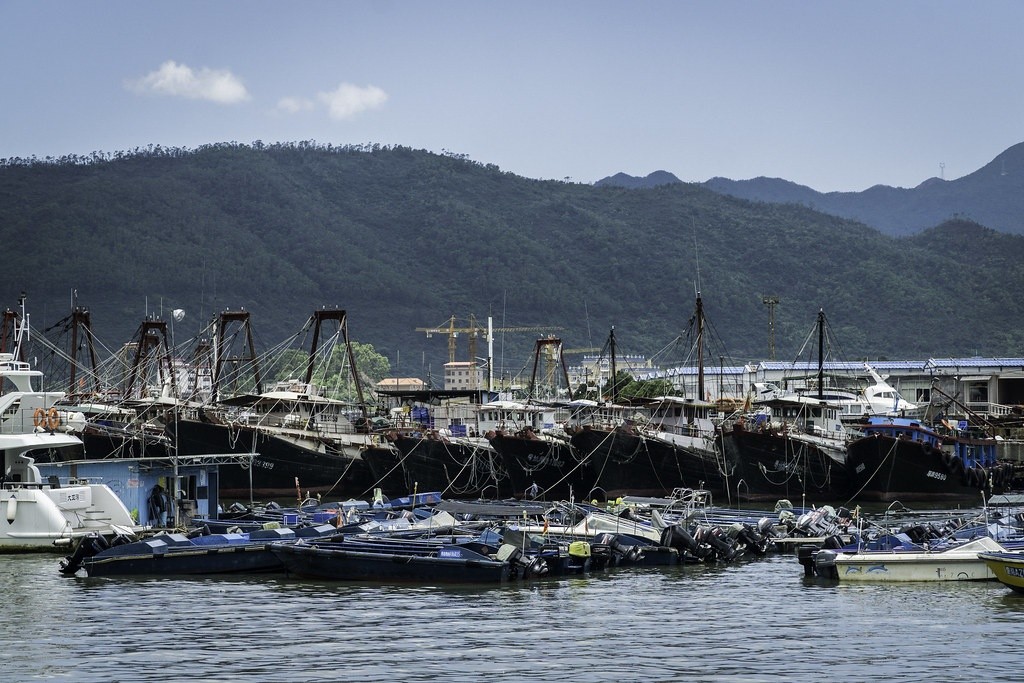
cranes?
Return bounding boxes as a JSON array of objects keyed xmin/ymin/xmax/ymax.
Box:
[{"xmin": 415, "ymin": 313, "xmax": 566, "ymax": 388}]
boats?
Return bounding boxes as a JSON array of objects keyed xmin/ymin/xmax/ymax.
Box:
[
  {"xmin": 61, "ymin": 471, "xmax": 1024, "ymax": 593},
  {"xmin": 0, "ymin": 293, "xmax": 134, "ymax": 553},
  {"xmin": 0, "ymin": 215, "xmax": 1024, "ymax": 507}
]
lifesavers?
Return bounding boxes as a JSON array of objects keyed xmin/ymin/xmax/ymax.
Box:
[
  {"xmin": 48, "ymin": 408, "xmax": 59, "ymax": 430},
  {"xmin": 34, "ymin": 409, "xmax": 46, "ymax": 427}
]
